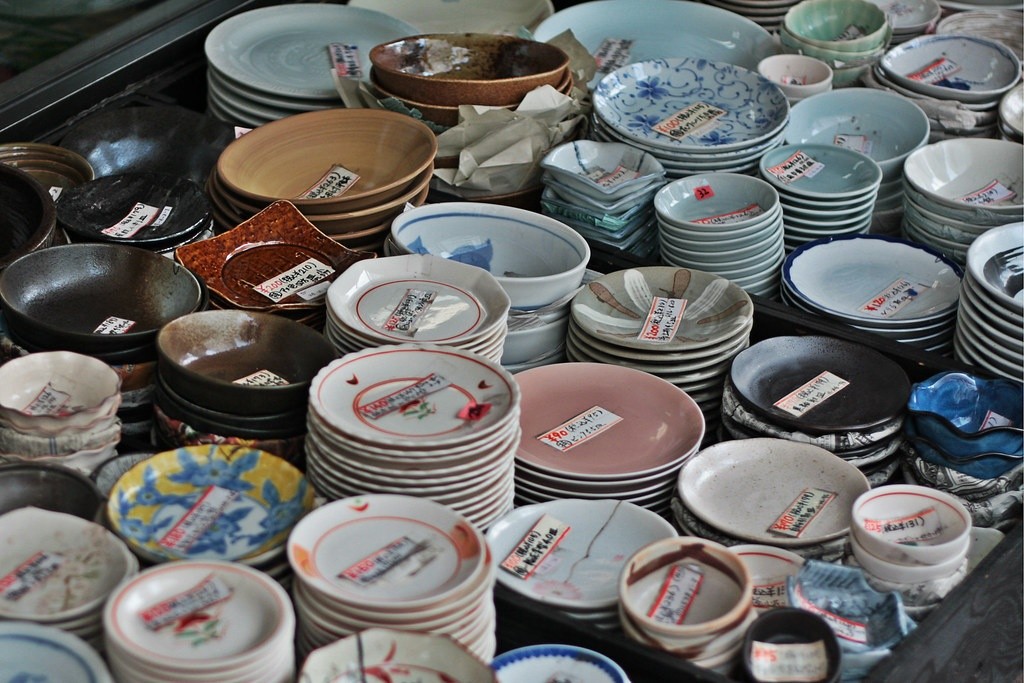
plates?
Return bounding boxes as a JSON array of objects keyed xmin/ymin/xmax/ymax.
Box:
[{"xmin": 0, "ymin": 0, "xmax": 1024, "ymax": 683}]
